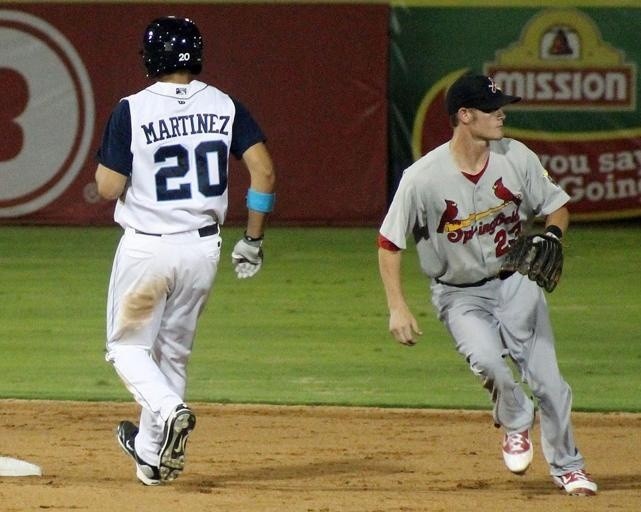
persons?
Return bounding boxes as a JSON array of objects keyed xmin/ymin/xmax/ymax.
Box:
[
  {"xmin": 94, "ymin": 16, "xmax": 277, "ymax": 486},
  {"xmin": 377, "ymin": 75, "xmax": 600, "ymax": 497}
]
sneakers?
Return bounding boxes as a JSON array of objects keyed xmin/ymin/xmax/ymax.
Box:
[
  {"xmin": 115, "ymin": 419, "xmax": 161, "ymax": 486},
  {"xmin": 157, "ymin": 403, "xmax": 196, "ymax": 481},
  {"xmin": 503, "ymin": 426, "xmax": 534, "ymax": 474},
  {"xmin": 552, "ymin": 467, "xmax": 597, "ymax": 495}
]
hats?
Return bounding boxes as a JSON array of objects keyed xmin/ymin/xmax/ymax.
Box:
[{"xmin": 445, "ymin": 72, "xmax": 522, "ymax": 116}]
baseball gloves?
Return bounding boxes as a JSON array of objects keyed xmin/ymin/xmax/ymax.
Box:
[{"xmin": 499, "ymin": 234, "xmax": 563, "ymax": 293}]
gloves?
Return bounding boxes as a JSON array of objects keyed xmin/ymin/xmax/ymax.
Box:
[{"xmin": 231, "ymin": 235, "xmax": 264, "ymax": 279}]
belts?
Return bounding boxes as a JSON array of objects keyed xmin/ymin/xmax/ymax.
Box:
[
  {"xmin": 134, "ymin": 222, "xmax": 219, "ymax": 238},
  {"xmin": 437, "ymin": 269, "xmax": 517, "ymax": 288}
]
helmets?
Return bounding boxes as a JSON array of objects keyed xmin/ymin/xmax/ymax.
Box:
[{"xmin": 142, "ymin": 16, "xmax": 203, "ymax": 78}]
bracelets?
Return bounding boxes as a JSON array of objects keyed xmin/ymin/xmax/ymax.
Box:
[
  {"xmin": 245, "ymin": 230, "xmax": 265, "ymax": 242},
  {"xmin": 544, "ymin": 224, "xmax": 564, "ymax": 239}
]
